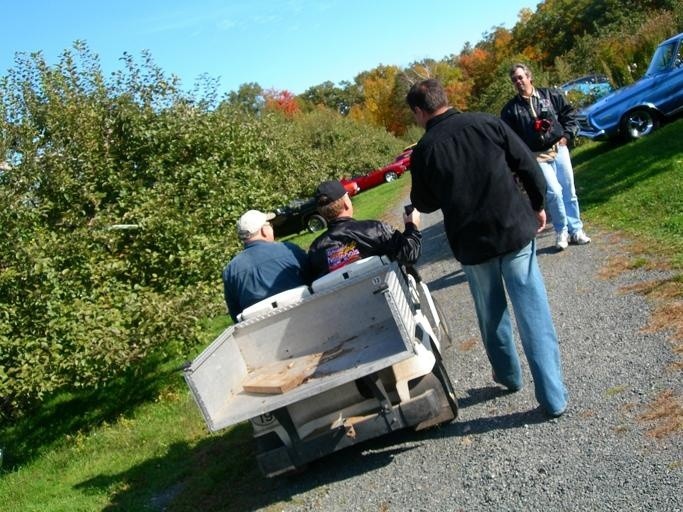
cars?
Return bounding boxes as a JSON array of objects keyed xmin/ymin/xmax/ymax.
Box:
[
  {"xmin": 575, "ymin": 32, "xmax": 683, "ymax": 141},
  {"xmin": 559, "ymin": 76, "xmax": 611, "ymax": 99},
  {"xmin": 267, "ymin": 198, "xmax": 328, "ymax": 239},
  {"xmin": 339, "ymin": 142, "xmax": 418, "ymax": 197}
]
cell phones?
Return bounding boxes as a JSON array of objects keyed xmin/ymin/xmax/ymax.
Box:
[{"xmin": 404, "ymin": 204, "xmax": 414, "ymax": 216}]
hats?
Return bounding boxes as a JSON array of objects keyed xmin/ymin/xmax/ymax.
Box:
[
  {"xmin": 315, "ymin": 180, "xmax": 347, "ymax": 208},
  {"xmin": 236, "ymin": 209, "xmax": 276, "ymax": 235}
]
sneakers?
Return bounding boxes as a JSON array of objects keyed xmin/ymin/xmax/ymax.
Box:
[
  {"xmin": 555, "ymin": 232, "xmax": 571, "ymax": 249},
  {"xmin": 571, "ymin": 230, "xmax": 591, "ymax": 245}
]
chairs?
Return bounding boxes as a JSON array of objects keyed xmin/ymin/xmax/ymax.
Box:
[
  {"xmin": 312, "ymin": 255, "xmax": 420, "ymax": 307},
  {"xmin": 236, "ymin": 285, "xmax": 311, "ymax": 322}
]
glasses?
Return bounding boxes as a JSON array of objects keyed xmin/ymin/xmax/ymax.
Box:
[{"xmin": 511, "ymin": 74, "xmax": 524, "ymax": 83}]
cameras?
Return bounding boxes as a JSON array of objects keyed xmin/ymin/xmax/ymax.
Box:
[{"xmin": 541, "ymin": 119, "xmax": 550, "ymax": 134}]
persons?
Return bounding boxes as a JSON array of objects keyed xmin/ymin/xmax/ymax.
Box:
[
  {"xmin": 309, "ymin": 180, "xmax": 421, "ymax": 396},
  {"xmin": 407, "ymin": 78, "xmax": 566, "ymax": 419},
  {"xmin": 500, "ymin": 63, "xmax": 592, "ymax": 250},
  {"xmin": 221, "ymin": 209, "xmax": 313, "ymax": 332}
]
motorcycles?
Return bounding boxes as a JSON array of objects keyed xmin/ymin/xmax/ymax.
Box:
[{"xmin": 185, "ymin": 255, "xmax": 459, "ymax": 480}]
